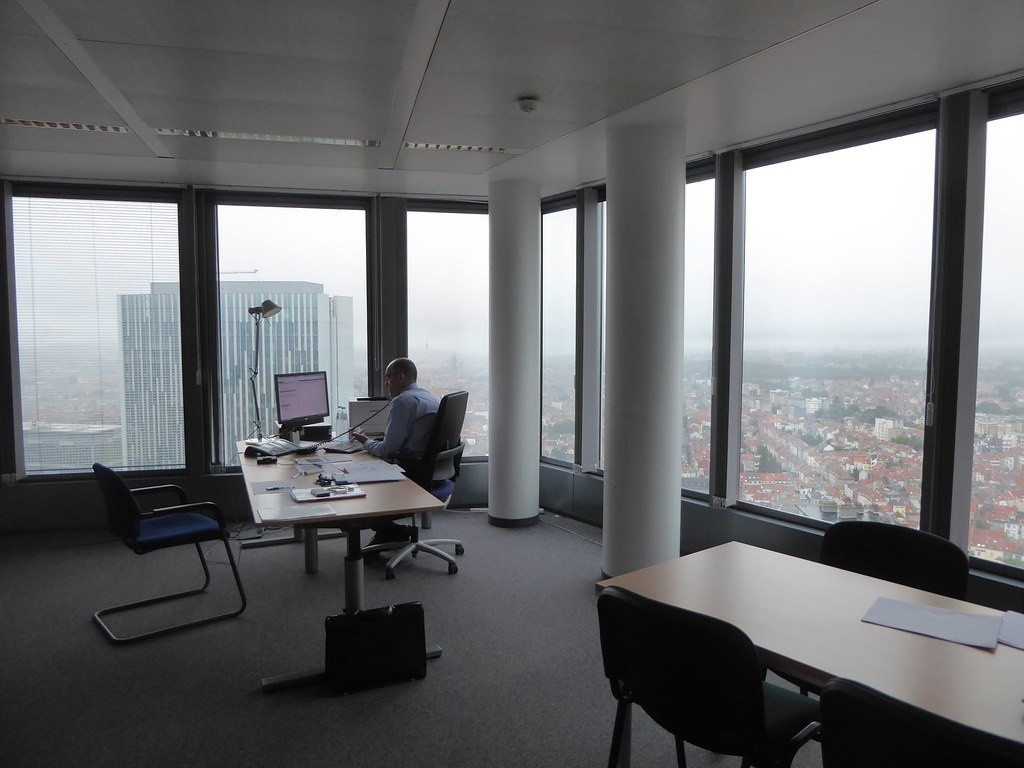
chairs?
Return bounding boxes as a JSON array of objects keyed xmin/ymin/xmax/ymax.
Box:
[
  {"xmin": 799, "ymin": 520, "xmax": 968, "ymax": 747},
  {"xmin": 90, "ymin": 464, "xmax": 247, "ymax": 643},
  {"xmin": 362, "ymin": 390, "xmax": 469, "ymax": 579},
  {"xmin": 596, "ymin": 586, "xmax": 1024, "ymax": 767}
]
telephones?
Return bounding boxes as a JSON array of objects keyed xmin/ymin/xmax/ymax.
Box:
[{"xmin": 292, "ymin": 442, "xmax": 318, "ymax": 453}]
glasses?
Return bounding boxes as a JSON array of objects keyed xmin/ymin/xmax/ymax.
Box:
[{"xmin": 385, "ymin": 372, "xmax": 406, "ymax": 381}]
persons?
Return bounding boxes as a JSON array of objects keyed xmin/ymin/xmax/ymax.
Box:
[{"xmin": 351, "ymin": 358, "xmax": 456, "ymax": 549}]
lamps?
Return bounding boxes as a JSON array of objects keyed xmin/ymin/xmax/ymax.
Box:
[{"xmin": 247, "ymin": 300, "xmax": 282, "ymax": 439}]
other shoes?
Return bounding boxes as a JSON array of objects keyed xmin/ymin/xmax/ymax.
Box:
[{"xmin": 365, "ymin": 525, "xmax": 410, "ymax": 546}]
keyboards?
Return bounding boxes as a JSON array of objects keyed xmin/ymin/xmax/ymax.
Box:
[{"xmin": 324, "ymin": 440, "xmax": 365, "ymax": 454}]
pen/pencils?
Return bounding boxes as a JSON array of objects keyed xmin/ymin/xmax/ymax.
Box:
[
  {"xmin": 266, "ymin": 486, "xmax": 295, "ymax": 490},
  {"xmin": 292, "ymin": 473, "xmax": 302, "ymax": 479}
]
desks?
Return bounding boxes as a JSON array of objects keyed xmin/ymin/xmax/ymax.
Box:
[
  {"xmin": 595, "ymin": 542, "xmax": 1024, "ymax": 768},
  {"xmin": 236, "ymin": 439, "xmax": 445, "ymax": 692}
]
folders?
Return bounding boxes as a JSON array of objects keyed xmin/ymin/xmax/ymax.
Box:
[{"xmin": 332, "ymin": 473, "xmax": 347, "ymax": 484}]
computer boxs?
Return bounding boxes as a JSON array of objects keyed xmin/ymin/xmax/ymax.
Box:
[{"xmin": 274, "ymin": 421, "xmax": 332, "ymax": 441}]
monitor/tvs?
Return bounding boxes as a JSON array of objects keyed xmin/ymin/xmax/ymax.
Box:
[{"xmin": 274, "ymin": 371, "xmax": 329, "ymax": 448}]
